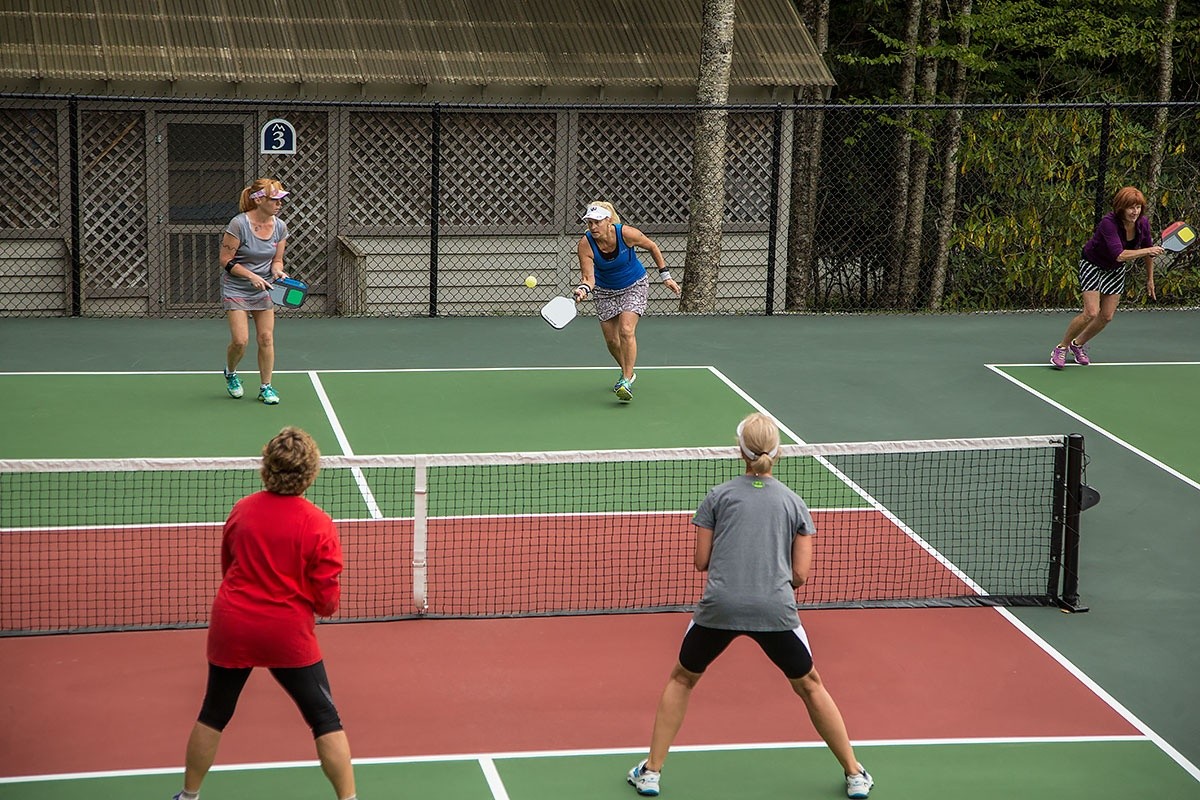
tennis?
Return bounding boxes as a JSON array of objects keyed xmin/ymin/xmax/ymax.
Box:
[{"xmin": 525, "ymin": 275, "xmax": 538, "ymax": 289}]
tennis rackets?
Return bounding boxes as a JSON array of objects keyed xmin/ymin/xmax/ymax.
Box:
[
  {"xmin": 1148, "ymin": 220, "xmax": 1197, "ymax": 259},
  {"xmin": 539, "ymin": 287, "xmax": 588, "ymax": 330},
  {"xmin": 250, "ymin": 276, "xmax": 309, "ymax": 309}
]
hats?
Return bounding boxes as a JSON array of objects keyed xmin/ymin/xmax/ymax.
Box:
[
  {"xmin": 735, "ymin": 418, "xmax": 778, "ymax": 461},
  {"xmin": 248, "ymin": 184, "xmax": 291, "ymax": 199},
  {"xmin": 581, "ymin": 206, "xmax": 612, "ymax": 222}
]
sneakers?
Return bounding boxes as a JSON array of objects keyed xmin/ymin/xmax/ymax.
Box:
[
  {"xmin": 842, "ymin": 762, "xmax": 874, "ymax": 800},
  {"xmin": 614, "ymin": 369, "xmax": 637, "ymax": 391},
  {"xmin": 223, "ymin": 366, "xmax": 245, "ymax": 399},
  {"xmin": 625, "ymin": 757, "xmax": 663, "ymax": 798},
  {"xmin": 1068, "ymin": 338, "xmax": 1090, "ymax": 367},
  {"xmin": 257, "ymin": 385, "xmax": 280, "ymax": 404},
  {"xmin": 1049, "ymin": 343, "xmax": 1070, "ymax": 370},
  {"xmin": 616, "ymin": 378, "xmax": 634, "ymax": 402}
]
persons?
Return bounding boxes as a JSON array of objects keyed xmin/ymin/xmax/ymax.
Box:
[
  {"xmin": 171, "ymin": 425, "xmax": 360, "ymax": 799},
  {"xmin": 220, "ymin": 179, "xmax": 289, "ymax": 405},
  {"xmin": 1050, "ymin": 186, "xmax": 1156, "ymax": 368},
  {"xmin": 626, "ymin": 413, "xmax": 874, "ymax": 799},
  {"xmin": 574, "ymin": 201, "xmax": 680, "ymax": 401}
]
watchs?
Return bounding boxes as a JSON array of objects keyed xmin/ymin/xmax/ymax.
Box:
[{"xmin": 659, "ymin": 266, "xmax": 669, "ymax": 273}]
